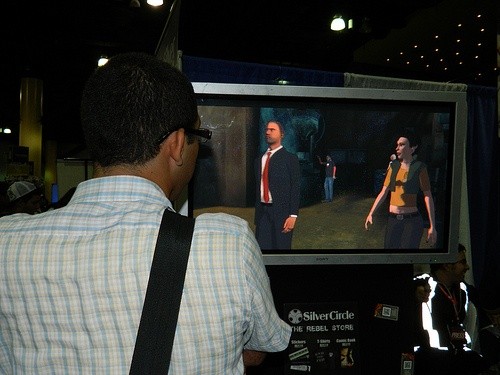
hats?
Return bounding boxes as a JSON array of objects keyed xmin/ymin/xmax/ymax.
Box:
[
  {"xmin": 24, "ymin": 176, "xmax": 44, "ymax": 188},
  {"xmin": 7, "ymin": 181, "xmax": 37, "ymax": 201}
]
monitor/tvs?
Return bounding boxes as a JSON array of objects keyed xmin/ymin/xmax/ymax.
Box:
[{"xmin": 172, "ymin": 82, "xmax": 467, "ymax": 265}]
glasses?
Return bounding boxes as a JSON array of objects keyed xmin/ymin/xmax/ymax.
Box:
[{"xmin": 156, "ymin": 128, "xmax": 212, "ymax": 144}]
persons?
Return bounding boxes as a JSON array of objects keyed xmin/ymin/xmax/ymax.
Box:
[
  {"xmin": 0, "ymin": 49, "xmax": 293, "ymax": 375},
  {"xmin": 415, "ymin": 242, "xmax": 487, "ymax": 375}
]
